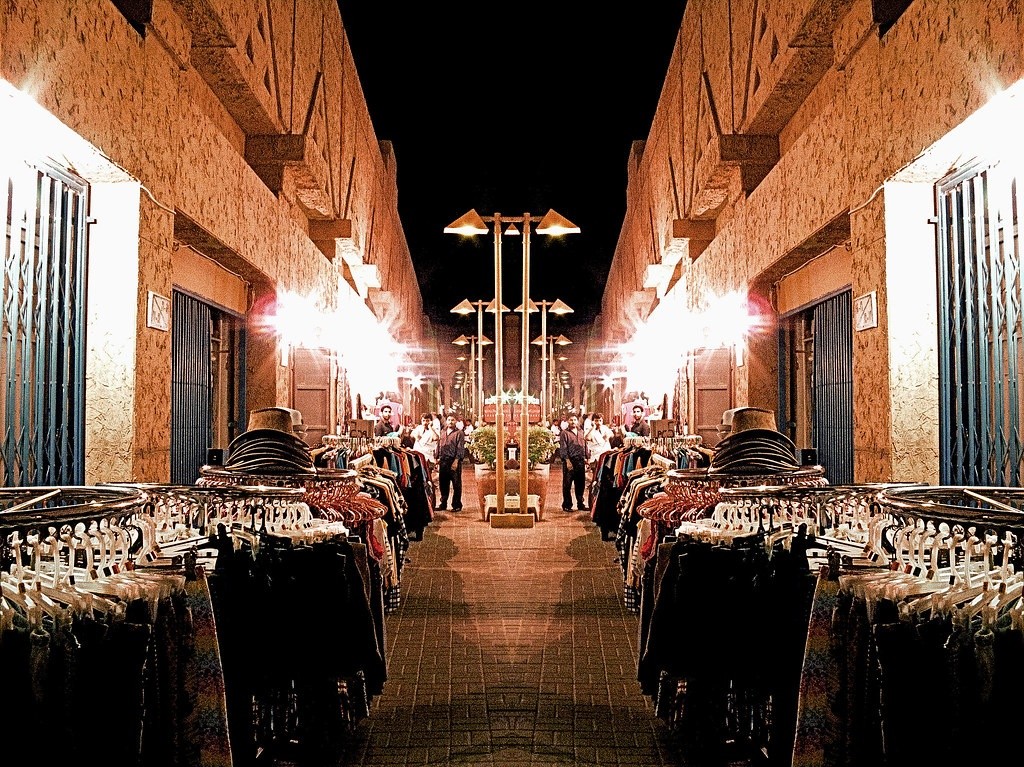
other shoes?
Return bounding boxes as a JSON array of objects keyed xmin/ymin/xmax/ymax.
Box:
[
  {"xmin": 578, "ymin": 506, "xmax": 590, "ymax": 511},
  {"xmin": 563, "ymin": 507, "xmax": 573, "ymax": 512},
  {"xmin": 435, "ymin": 507, "xmax": 446, "ymax": 511},
  {"xmin": 451, "ymin": 508, "xmax": 461, "ymax": 512}
]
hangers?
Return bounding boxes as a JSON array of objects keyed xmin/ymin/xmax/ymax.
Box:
[
  {"xmin": 0, "ymin": 436, "xmax": 404, "ymax": 636},
  {"xmin": 621, "ymin": 435, "xmax": 1024, "ymax": 640}
]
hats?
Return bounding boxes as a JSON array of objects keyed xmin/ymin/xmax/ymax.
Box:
[
  {"xmin": 225, "ymin": 407, "xmax": 318, "ymax": 476},
  {"xmin": 708, "ymin": 407, "xmax": 800, "ymax": 476}
]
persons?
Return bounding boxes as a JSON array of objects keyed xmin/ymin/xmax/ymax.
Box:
[
  {"xmin": 551, "ymin": 419, "xmax": 560, "ymax": 435},
  {"xmin": 372, "ymin": 404, "xmax": 394, "ymax": 468},
  {"xmin": 464, "ymin": 419, "xmax": 474, "ymax": 435},
  {"xmin": 559, "ymin": 414, "xmax": 588, "ymax": 512},
  {"xmin": 435, "ymin": 414, "xmax": 465, "ymax": 512},
  {"xmin": 410, "ymin": 412, "xmax": 440, "ymax": 475},
  {"xmin": 586, "ymin": 412, "xmax": 614, "ymax": 490},
  {"xmin": 456, "ymin": 414, "xmax": 464, "ymax": 431},
  {"xmin": 433, "ymin": 414, "xmax": 447, "ymax": 430},
  {"xmin": 560, "ymin": 414, "xmax": 588, "ymax": 431},
  {"xmin": 630, "ymin": 405, "xmax": 650, "ymax": 438}
]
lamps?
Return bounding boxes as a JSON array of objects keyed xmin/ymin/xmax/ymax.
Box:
[
  {"xmin": 453, "ymin": 352, "xmax": 573, "ymax": 389},
  {"xmin": 443, "ymin": 208, "xmax": 489, "ymax": 239},
  {"xmin": 548, "ymin": 299, "xmax": 574, "ymax": 315},
  {"xmin": 451, "ymin": 334, "xmax": 470, "ymax": 347},
  {"xmin": 530, "ymin": 335, "xmax": 549, "ymax": 346},
  {"xmin": 485, "ymin": 298, "xmax": 511, "ymax": 315},
  {"xmin": 514, "ymin": 298, "xmax": 539, "ymax": 313},
  {"xmin": 476, "ymin": 335, "xmax": 494, "ymax": 345},
  {"xmin": 555, "ymin": 334, "xmax": 573, "ymax": 345},
  {"xmin": 535, "ymin": 209, "xmax": 580, "ymax": 238},
  {"xmin": 450, "ymin": 298, "xmax": 477, "ymax": 315}
]
